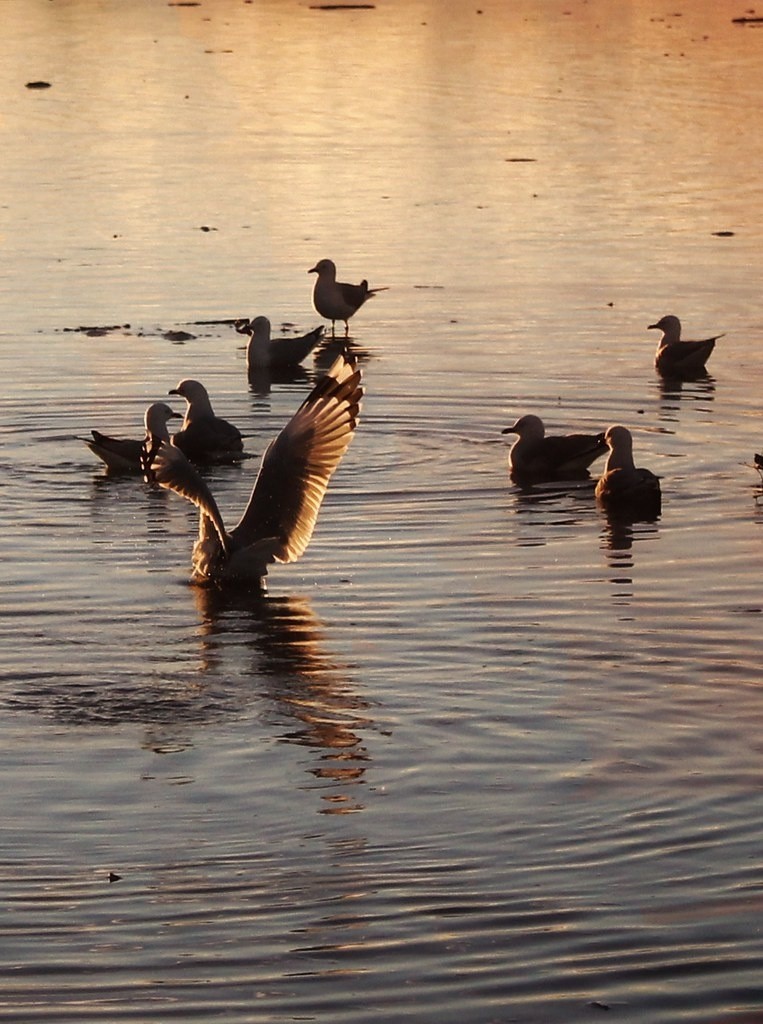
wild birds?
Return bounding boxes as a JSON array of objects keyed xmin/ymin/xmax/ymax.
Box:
[
  {"xmin": 647, "ymin": 314, "xmax": 727, "ymax": 380},
  {"xmin": 84, "ymin": 344, "xmax": 371, "ymax": 593},
  {"xmin": 307, "ymin": 258, "xmax": 391, "ymax": 341},
  {"xmin": 500, "ymin": 413, "xmax": 666, "ymax": 521},
  {"xmin": 231, "ymin": 314, "xmax": 327, "ymax": 385}
]
